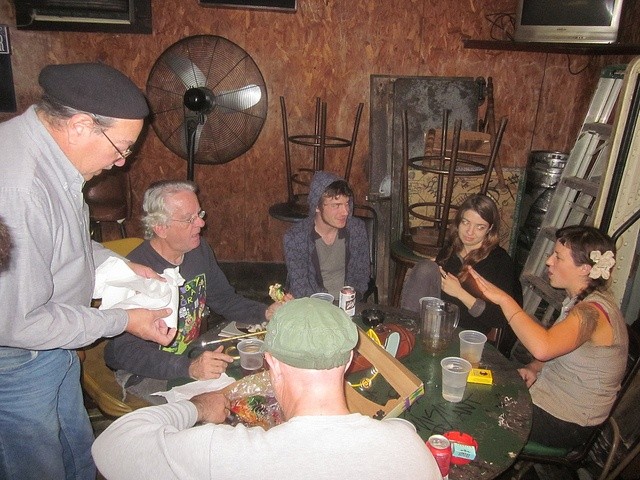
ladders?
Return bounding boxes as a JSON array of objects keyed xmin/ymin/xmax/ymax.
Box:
[{"xmin": 519, "ymin": 70, "xmax": 627, "ymax": 329}]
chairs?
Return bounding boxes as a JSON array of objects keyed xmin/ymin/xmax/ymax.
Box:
[
  {"xmin": 485, "ymin": 280, "xmax": 523, "ymax": 359},
  {"xmin": 521, "ymin": 322, "xmax": 639, "ymax": 479},
  {"xmin": 358, "ymin": 203, "xmax": 380, "ymax": 304},
  {"xmin": 80, "ymin": 237, "xmax": 153, "ymax": 418},
  {"xmin": 82, "ymin": 168, "xmax": 131, "ymax": 242}
]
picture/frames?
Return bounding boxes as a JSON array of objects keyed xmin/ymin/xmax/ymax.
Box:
[
  {"xmin": 197, "ymin": 0, "xmax": 297, "ymax": 12},
  {"xmin": 14, "ymin": 0, "xmax": 152, "ymax": 35}
]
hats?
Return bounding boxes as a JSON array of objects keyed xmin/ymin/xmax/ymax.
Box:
[
  {"xmin": 258, "ymin": 297, "xmax": 358, "ymax": 370},
  {"xmin": 38, "ymin": 63, "xmax": 150, "ymax": 119}
]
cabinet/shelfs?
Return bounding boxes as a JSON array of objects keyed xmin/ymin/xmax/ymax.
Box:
[{"xmin": 463, "ymin": 39, "xmax": 640, "ymax": 55}]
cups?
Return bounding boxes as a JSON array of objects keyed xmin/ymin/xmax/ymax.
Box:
[
  {"xmin": 458, "ymin": 330, "xmax": 487, "ymax": 365},
  {"xmin": 236, "ymin": 338, "xmax": 266, "ymax": 371},
  {"xmin": 440, "ymin": 356, "xmax": 472, "ymax": 403},
  {"xmin": 309, "ymin": 293, "xmax": 335, "ymax": 305}
]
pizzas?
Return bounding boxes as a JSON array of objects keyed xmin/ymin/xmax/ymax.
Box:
[
  {"xmin": 213, "ymin": 392, "xmax": 288, "ymax": 431},
  {"xmin": 268, "ymin": 283, "xmax": 288, "ymax": 303}
]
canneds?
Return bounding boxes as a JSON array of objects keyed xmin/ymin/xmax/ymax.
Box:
[
  {"xmin": 338, "ymin": 285, "xmax": 356, "ymax": 319},
  {"xmin": 426, "ymin": 434, "xmax": 451, "ymax": 480}
]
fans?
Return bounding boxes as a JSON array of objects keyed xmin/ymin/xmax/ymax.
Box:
[{"xmin": 145, "ymin": 35, "xmax": 268, "ymax": 182}]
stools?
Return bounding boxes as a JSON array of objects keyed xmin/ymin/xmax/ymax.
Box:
[
  {"xmin": 401, "ymin": 107, "xmax": 508, "ymax": 260},
  {"xmin": 279, "ymin": 95, "xmax": 363, "ymax": 216}
]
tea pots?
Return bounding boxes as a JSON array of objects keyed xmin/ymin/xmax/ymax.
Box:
[{"xmin": 421, "ymin": 298, "xmax": 461, "ymax": 353}]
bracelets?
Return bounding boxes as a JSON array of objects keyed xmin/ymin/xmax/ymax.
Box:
[{"xmin": 508, "ymin": 310, "xmax": 524, "ymax": 326}]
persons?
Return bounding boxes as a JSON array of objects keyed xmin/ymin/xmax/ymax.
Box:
[
  {"xmin": 105, "ymin": 182, "xmax": 294, "ymax": 406},
  {"xmin": 0, "ymin": 63, "xmax": 177, "ymax": 480},
  {"xmin": 468, "ymin": 226, "xmax": 629, "ymax": 449},
  {"xmin": 283, "ymin": 171, "xmax": 371, "ymax": 302},
  {"xmin": 399, "ymin": 194, "xmax": 523, "ymax": 358},
  {"xmin": 91, "ymin": 297, "xmax": 443, "ymax": 480}
]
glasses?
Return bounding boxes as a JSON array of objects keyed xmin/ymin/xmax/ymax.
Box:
[
  {"xmin": 170, "ymin": 211, "xmax": 205, "ymax": 224},
  {"xmin": 100, "ymin": 128, "xmax": 132, "ymax": 160}
]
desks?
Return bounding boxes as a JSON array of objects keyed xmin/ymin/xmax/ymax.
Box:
[
  {"xmin": 389, "ymin": 240, "xmax": 416, "ymax": 303},
  {"xmin": 271, "ymin": 203, "xmax": 307, "ymax": 224}
]
